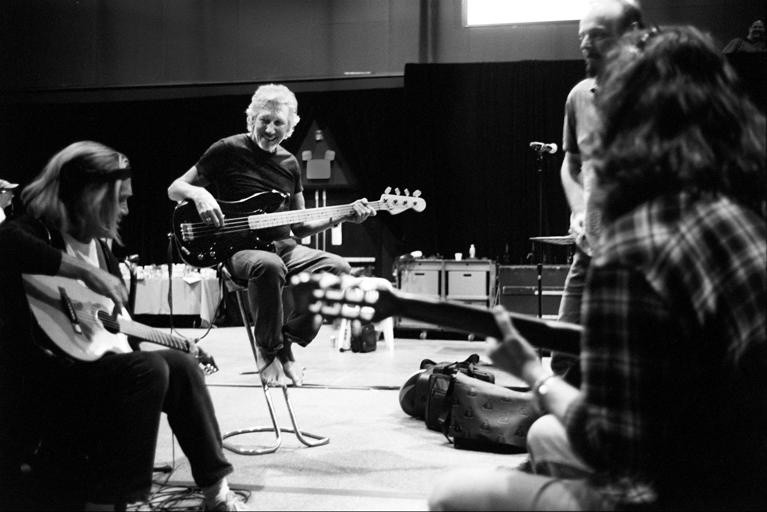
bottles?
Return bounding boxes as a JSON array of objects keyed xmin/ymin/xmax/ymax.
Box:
[{"xmin": 469, "ymin": 244, "xmax": 475, "ymax": 259}]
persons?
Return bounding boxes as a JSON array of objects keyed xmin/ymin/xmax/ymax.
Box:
[
  {"xmin": 427, "ymin": 24, "xmax": 765, "ymax": 511},
  {"xmin": 719, "ymin": 20, "xmax": 766, "ymax": 117},
  {"xmin": 0, "ymin": 141, "xmax": 247, "ymax": 510},
  {"xmin": 0, "ymin": 177, "xmax": 19, "ymax": 225},
  {"xmin": 168, "ymin": 84, "xmax": 379, "ymax": 387},
  {"xmin": 550, "ymin": 3, "xmax": 647, "ymax": 390}
]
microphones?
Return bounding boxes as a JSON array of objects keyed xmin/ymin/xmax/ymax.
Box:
[{"xmin": 530, "ymin": 142, "xmax": 558, "ymax": 154}]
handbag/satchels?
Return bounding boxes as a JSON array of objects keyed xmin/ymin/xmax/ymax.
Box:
[{"xmin": 399, "ymin": 353, "xmax": 546, "ymax": 451}]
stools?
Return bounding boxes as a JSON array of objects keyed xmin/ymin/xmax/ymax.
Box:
[{"xmin": 216, "ymin": 263, "xmax": 329, "ymax": 456}]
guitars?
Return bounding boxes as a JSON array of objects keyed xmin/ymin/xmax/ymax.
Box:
[
  {"xmin": 19, "ymin": 271, "xmax": 218, "ymax": 375},
  {"xmin": 286, "ymin": 271, "xmax": 587, "ymax": 360},
  {"xmin": 172, "ymin": 185, "xmax": 426, "ymax": 269}
]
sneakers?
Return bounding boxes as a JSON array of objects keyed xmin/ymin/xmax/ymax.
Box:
[{"xmin": 200, "ymin": 489, "xmax": 253, "ymax": 512}]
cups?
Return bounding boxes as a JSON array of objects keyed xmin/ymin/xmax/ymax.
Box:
[{"xmin": 455, "ymin": 253, "xmax": 462, "ymax": 261}]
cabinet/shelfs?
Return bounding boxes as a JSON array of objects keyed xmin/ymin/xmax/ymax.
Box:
[{"xmin": 394, "ymin": 258, "xmax": 495, "ymax": 341}]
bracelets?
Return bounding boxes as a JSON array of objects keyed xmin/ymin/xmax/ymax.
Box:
[
  {"xmin": 532, "ymin": 374, "xmax": 559, "ymax": 396},
  {"xmin": 329, "ymin": 217, "xmax": 338, "ymax": 229}
]
